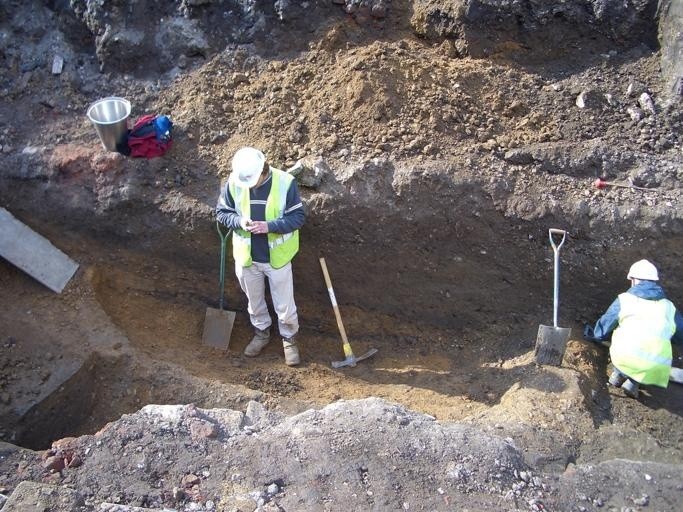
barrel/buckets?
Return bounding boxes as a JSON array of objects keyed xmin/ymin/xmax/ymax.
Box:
[{"xmin": 87, "ymin": 97, "xmax": 132, "ymax": 151}]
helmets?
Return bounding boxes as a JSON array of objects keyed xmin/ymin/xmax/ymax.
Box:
[
  {"xmin": 627, "ymin": 259, "xmax": 660, "ymax": 281},
  {"xmin": 230, "ymin": 146, "xmax": 266, "ymax": 190}
]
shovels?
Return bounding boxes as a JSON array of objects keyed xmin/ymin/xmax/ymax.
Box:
[
  {"xmin": 533, "ymin": 228, "xmax": 572, "ymax": 366},
  {"xmin": 202, "ymin": 221, "xmax": 237, "ymax": 351}
]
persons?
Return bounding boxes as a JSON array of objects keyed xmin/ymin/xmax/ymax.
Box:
[
  {"xmin": 592, "ymin": 259, "xmax": 682, "ymax": 398},
  {"xmin": 215, "ymin": 147, "xmax": 305, "ymax": 367}
]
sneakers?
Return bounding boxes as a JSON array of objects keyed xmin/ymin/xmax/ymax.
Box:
[
  {"xmin": 621, "ymin": 376, "xmax": 640, "ymax": 399},
  {"xmin": 608, "ymin": 367, "xmax": 625, "ymax": 387}
]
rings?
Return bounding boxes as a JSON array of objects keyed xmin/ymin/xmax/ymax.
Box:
[{"xmin": 257, "ymin": 231, "xmax": 261, "ymax": 234}]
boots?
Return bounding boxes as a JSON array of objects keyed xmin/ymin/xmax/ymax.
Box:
[
  {"xmin": 281, "ymin": 333, "xmax": 302, "ymax": 366},
  {"xmin": 242, "ymin": 327, "xmax": 270, "ymax": 358}
]
